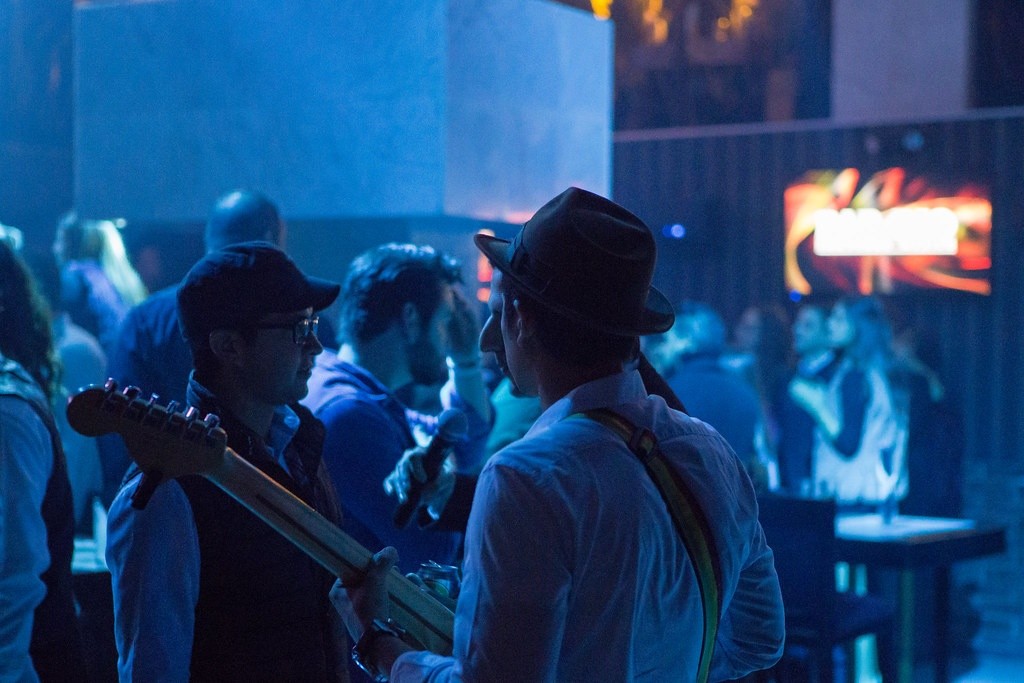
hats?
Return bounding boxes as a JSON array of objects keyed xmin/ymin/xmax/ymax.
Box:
[
  {"xmin": 177, "ymin": 238, "xmax": 339, "ymax": 347},
  {"xmin": 474, "ymin": 187, "xmax": 674, "ymax": 338}
]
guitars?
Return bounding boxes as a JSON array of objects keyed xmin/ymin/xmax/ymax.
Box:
[{"xmin": 66, "ymin": 377, "xmax": 458, "ymax": 658}]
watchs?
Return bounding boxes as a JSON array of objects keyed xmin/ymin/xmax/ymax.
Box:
[{"xmin": 352, "ymin": 619, "xmax": 398, "ymax": 683}]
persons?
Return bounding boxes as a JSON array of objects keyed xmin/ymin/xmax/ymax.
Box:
[{"xmin": 0, "ymin": 187, "xmax": 962, "ymax": 683}]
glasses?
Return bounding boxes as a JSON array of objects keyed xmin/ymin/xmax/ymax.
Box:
[{"xmin": 255, "ymin": 315, "xmax": 321, "ymax": 345}]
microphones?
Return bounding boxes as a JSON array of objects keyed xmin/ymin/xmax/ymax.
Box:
[{"xmin": 393, "ymin": 408, "xmax": 470, "ymax": 526}]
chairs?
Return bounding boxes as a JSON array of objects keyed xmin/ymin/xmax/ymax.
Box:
[{"xmin": 738, "ymin": 494, "xmax": 897, "ymax": 683}]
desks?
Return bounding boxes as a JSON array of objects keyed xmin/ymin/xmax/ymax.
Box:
[{"xmin": 834, "ymin": 511, "xmax": 1009, "ymax": 683}]
